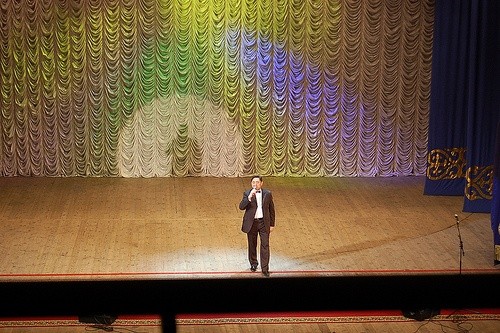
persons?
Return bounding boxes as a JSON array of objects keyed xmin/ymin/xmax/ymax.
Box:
[{"xmin": 239, "ymin": 176, "xmax": 275, "ymax": 276}]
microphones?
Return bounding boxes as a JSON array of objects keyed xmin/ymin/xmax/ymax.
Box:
[
  {"xmin": 454, "ymin": 214, "xmax": 459, "ymax": 224},
  {"xmin": 253, "ymin": 187, "xmax": 256, "ymax": 195}
]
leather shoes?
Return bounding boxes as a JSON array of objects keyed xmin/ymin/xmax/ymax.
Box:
[
  {"xmin": 263, "ymin": 271, "xmax": 270, "ymax": 276},
  {"xmin": 250, "ymin": 265, "xmax": 256, "ymax": 272}
]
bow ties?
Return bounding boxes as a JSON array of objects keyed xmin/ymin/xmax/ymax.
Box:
[{"xmin": 256, "ymin": 191, "xmax": 261, "ymax": 193}]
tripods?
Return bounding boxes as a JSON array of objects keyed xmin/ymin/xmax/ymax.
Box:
[{"xmin": 446, "ymin": 223, "xmax": 480, "ymax": 318}]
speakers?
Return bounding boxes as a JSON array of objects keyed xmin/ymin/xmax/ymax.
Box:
[
  {"xmin": 79, "ymin": 314, "xmax": 116, "ymax": 325},
  {"xmin": 403, "ymin": 307, "xmax": 440, "ymax": 321}
]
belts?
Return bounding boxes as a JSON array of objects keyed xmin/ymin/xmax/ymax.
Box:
[{"xmin": 254, "ymin": 218, "xmax": 263, "ymax": 220}]
